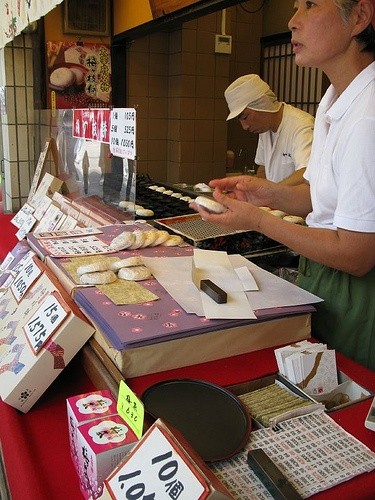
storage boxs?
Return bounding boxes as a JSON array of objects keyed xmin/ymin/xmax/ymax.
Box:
[
  {"xmin": 0, "ymin": 242, "xmax": 95, "ymax": 415},
  {"xmin": 27, "ymin": 222, "xmax": 316, "ymax": 379},
  {"xmin": 66, "ymin": 389, "xmax": 136, "ymax": 500}
]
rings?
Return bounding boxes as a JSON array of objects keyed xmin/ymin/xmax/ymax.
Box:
[{"xmin": 201, "ymin": 218, "xmax": 205, "ymax": 222}]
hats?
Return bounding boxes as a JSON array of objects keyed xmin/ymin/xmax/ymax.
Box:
[{"xmin": 223, "ymin": 74, "xmax": 282, "ymax": 121}]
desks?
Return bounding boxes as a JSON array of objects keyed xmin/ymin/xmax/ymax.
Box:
[{"xmin": 0, "ymin": 213, "xmax": 375, "ymax": 500}]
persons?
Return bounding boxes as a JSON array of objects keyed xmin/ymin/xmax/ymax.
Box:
[
  {"xmin": 188, "ymin": 0, "xmax": 375, "ymax": 372},
  {"xmin": 224, "ymin": 74, "xmax": 316, "ymax": 187},
  {"xmin": 61, "ymin": 110, "xmax": 134, "ymax": 206}
]
